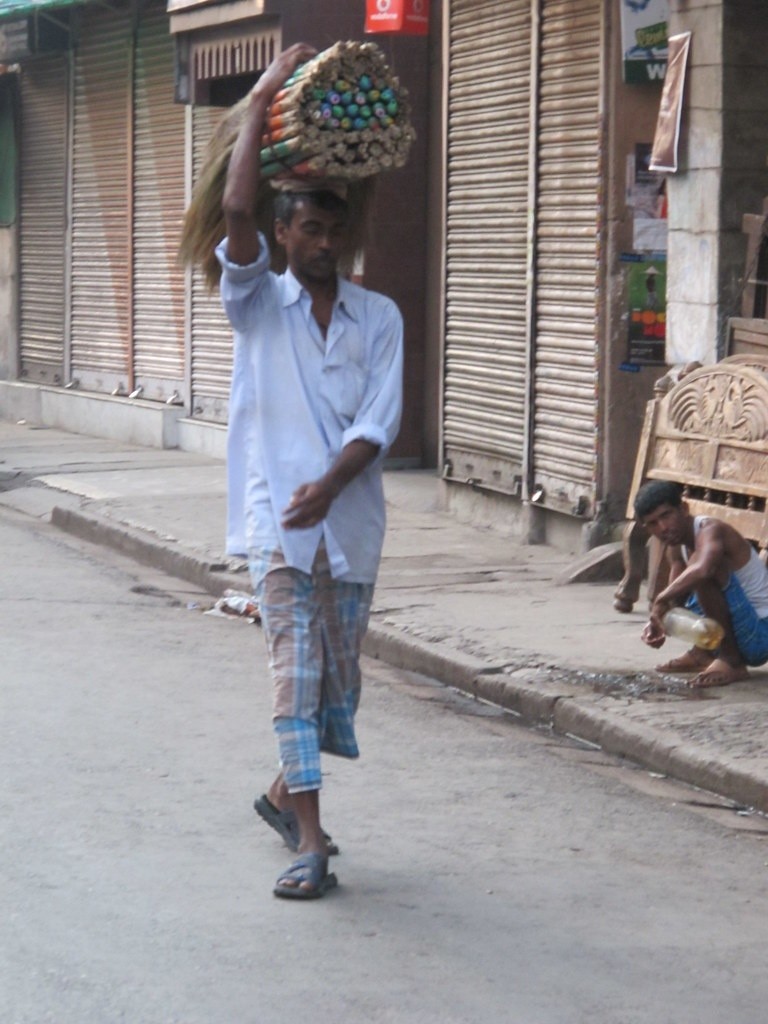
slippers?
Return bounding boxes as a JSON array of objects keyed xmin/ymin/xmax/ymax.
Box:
[
  {"xmin": 689, "ymin": 658, "xmax": 750, "ymax": 686},
  {"xmin": 655, "ymin": 649, "xmax": 712, "ymax": 672},
  {"xmin": 274, "ymin": 853, "xmax": 337, "ymax": 899},
  {"xmin": 255, "ymin": 793, "xmax": 340, "ymax": 856}
]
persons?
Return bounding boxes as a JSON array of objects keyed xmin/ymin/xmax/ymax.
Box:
[
  {"xmin": 211, "ymin": 39, "xmax": 407, "ymax": 904},
  {"xmin": 633, "ymin": 479, "xmax": 768, "ymax": 689}
]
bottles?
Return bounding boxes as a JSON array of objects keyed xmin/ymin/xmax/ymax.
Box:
[{"xmin": 659, "ymin": 605, "xmax": 724, "ymax": 649}]
